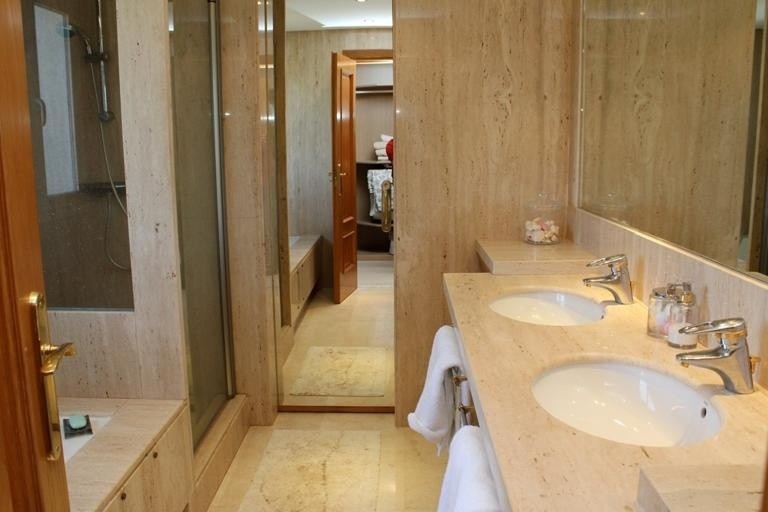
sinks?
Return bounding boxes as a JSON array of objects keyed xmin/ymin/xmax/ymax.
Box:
[
  {"xmin": 530, "ymin": 351, "xmax": 727, "ymax": 447},
  {"xmin": 487, "ymin": 286, "xmax": 606, "ymax": 326}
]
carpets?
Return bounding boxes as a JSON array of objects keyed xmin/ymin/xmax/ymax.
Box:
[
  {"xmin": 238, "ymin": 428, "xmax": 382, "ymax": 512},
  {"xmin": 288, "ymin": 345, "xmax": 389, "ymax": 398}
]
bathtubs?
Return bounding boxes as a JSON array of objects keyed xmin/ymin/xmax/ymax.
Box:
[{"xmin": 59, "ymin": 416, "xmax": 108, "ymax": 465}]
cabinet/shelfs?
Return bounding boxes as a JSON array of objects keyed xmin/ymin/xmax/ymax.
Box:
[
  {"xmin": 104, "ymin": 400, "xmax": 192, "ymax": 512},
  {"xmin": 288, "ymin": 234, "xmax": 322, "ymax": 325}
]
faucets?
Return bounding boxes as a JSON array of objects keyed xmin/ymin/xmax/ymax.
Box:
[
  {"xmin": 581, "ymin": 253, "xmax": 633, "ymax": 306},
  {"xmin": 675, "ymin": 317, "xmax": 754, "ymax": 393}
]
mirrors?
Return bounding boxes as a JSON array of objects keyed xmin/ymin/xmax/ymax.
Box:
[
  {"xmin": 275, "ymin": 0, "xmax": 397, "ymax": 414},
  {"xmin": 570, "ymin": 0, "xmax": 768, "ymax": 292}
]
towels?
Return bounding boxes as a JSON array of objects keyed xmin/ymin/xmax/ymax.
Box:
[
  {"xmin": 405, "ymin": 324, "xmax": 509, "ymax": 512},
  {"xmin": 366, "ymin": 167, "xmax": 394, "ymax": 219}
]
